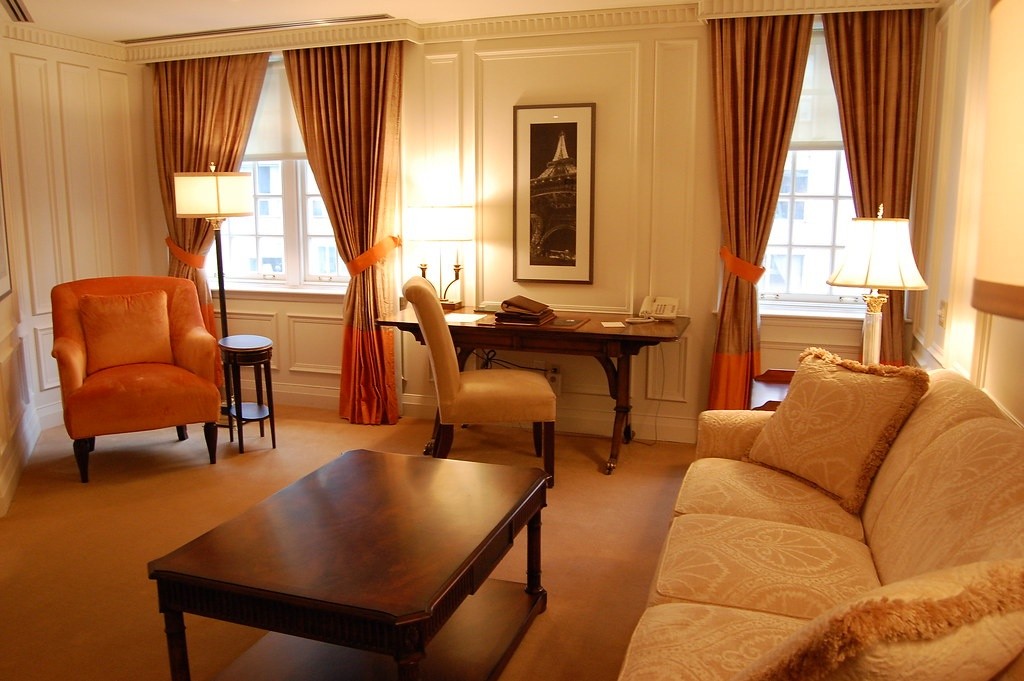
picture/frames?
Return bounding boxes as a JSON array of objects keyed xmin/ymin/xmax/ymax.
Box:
[{"xmin": 513, "ymin": 102, "xmax": 595, "ymax": 283}]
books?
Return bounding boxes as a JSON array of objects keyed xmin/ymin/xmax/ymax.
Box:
[{"xmin": 495, "ymin": 294, "xmax": 557, "ymax": 326}]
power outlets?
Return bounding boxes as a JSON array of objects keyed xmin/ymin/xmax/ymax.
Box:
[{"xmin": 544, "ymin": 361, "xmax": 561, "ymax": 379}]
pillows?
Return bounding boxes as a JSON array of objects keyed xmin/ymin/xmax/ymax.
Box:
[
  {"xmin": 79, "ymin": 289, "xmax": 173, "ymax": 376},
  {"xmin": 732, "ymin": 557, "xmax": 1024, "ymax": 681},
  {"xmin": 742, "ymin": 346, "xmax": 929, "ymax": 516}
]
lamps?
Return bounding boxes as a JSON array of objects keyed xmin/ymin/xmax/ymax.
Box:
[
  {"xmin": 826, "ymin": 203, "xmax": 929, "ymax": 366},
  {"xmin": 406, "ymin": 204, "xmax": 476, "ymax": 309},
  {"xmin": 173, "ymin": 160, "xmax": 254, "ymax": 427}
]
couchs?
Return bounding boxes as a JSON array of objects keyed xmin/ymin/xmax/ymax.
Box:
[
  {"xmin": 618, "ymin": 370, "xmax": 1024, "ymax": 679},
  {"xmin": 51, "ymin": 275, "xmax": 222, "ymax": 483}
]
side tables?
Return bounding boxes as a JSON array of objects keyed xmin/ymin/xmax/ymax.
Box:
[
  {"xmin": 750, "ymin": 368, "xmax": 796, "ymax": 410},
  {"xmin": 219, "ymin": 334, "xmax": 277, "ymax": 453}
]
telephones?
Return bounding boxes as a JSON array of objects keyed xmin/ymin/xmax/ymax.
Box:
[{"xmin": 638, "ymin": 296, "xmax": 680, "ymax": 322}]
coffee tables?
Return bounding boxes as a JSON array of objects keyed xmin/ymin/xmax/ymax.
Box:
[{"xmin": 146, "ymin": 447, "xmax": 547, "ymax": 681}]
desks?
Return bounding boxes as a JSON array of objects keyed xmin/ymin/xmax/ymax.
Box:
[{"xmin": 375, "ymin": 304, "xmax": 691, "ymax": 475}]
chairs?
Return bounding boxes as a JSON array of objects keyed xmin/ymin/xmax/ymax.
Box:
[{"xmin": 402, "ymin": 275, "xmax": 556, "ymax": 487}]
